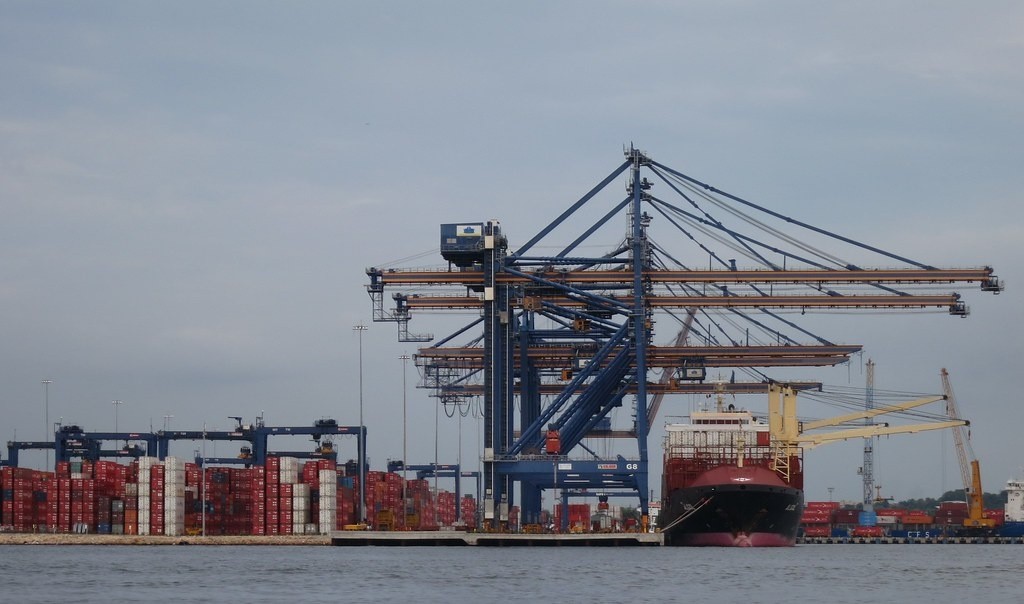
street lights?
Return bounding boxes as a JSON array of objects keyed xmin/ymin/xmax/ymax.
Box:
[
  {"xmin": 165, "ymin": 415, "xmax": 174, "ymax": 430},
  {"xmin": 827, "ymin": 487, "xmax": 834, "ymax": 502},
  {"xmin": 399, "ymin": 355, "xmax": 411, "ymax": 530},
  {"xmin": 353, "ymin": 325, "xmax": 369, "ymax": 521},
  {"xmin": 42, "ymin": 379, "xmax": 53, "ymax": 469},
  {"xmin": 112, "ymin": 401, "xmax": 123, "ymax": 462}
]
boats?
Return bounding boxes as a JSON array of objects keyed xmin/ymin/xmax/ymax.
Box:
[{"xmin": 660, "ymin": 374, "xmax": 805, "ymax": 547}]
